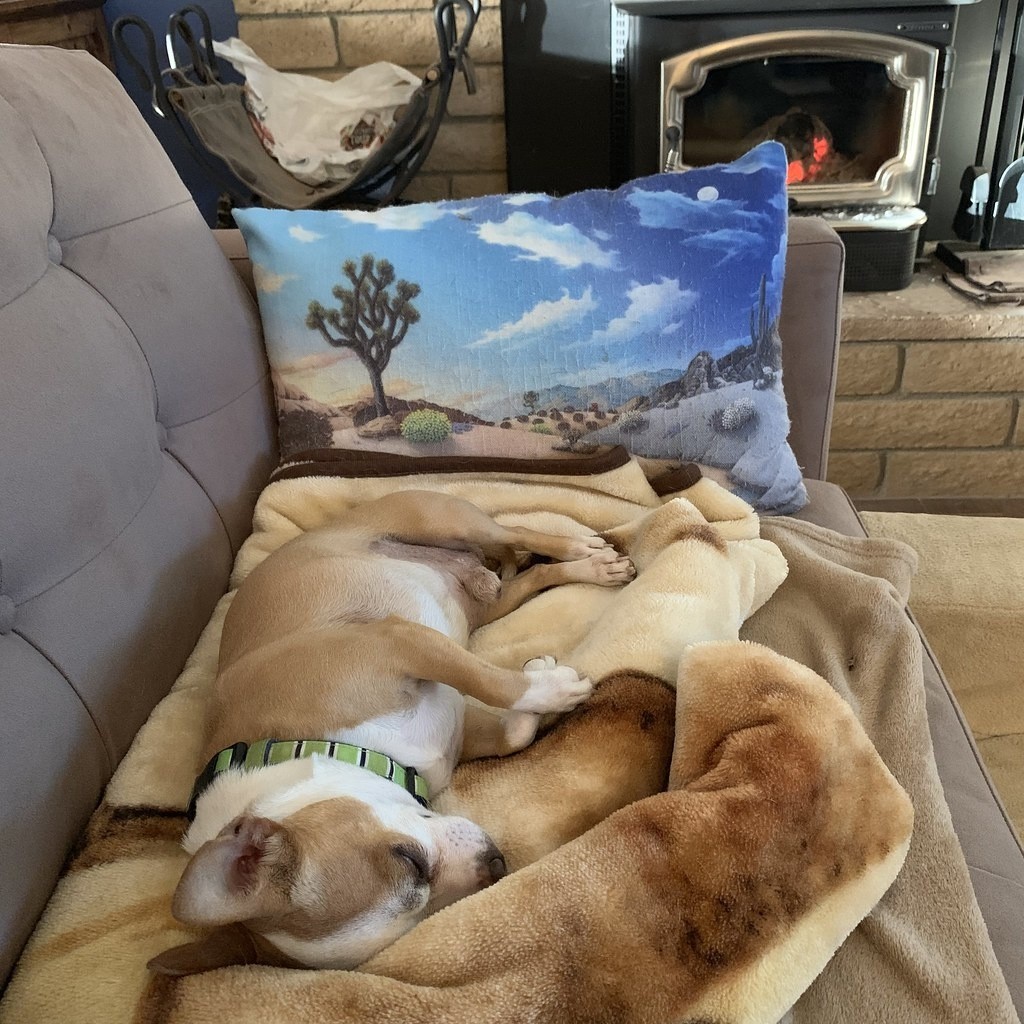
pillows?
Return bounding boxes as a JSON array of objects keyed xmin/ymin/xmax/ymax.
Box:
[{"xmin": 228, "ymin": 145, "xmax": 815, "ymax": 530}]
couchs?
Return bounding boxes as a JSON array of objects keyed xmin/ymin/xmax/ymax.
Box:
[{"xmin": 2, "ymin": 37, "xmax": 1018, "ymax": 1024}]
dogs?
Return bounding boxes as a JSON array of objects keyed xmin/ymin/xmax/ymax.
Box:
[{"xmin": 145, "ymin": 488, "xmax": 639, "ymax": 980}]
